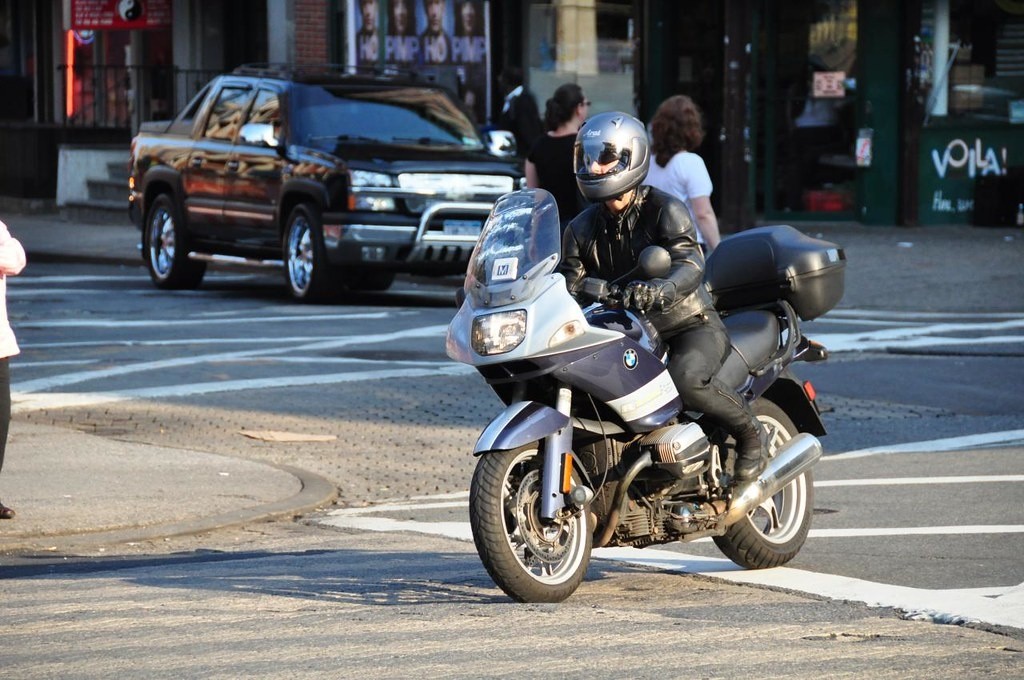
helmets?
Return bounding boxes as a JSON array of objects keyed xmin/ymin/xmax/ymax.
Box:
[{"xmin": 573, "ymin": 111, "xmax": 650, "ymax": 202}]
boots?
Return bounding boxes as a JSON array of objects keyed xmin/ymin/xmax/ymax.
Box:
[{"xmin": 732, "ymin": 415, "xmax": 768, "ymax": 480}]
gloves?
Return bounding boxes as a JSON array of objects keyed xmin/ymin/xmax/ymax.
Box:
[{"xmin": 621, "ymin": 280, "xmax": 656, "ymax": 312}]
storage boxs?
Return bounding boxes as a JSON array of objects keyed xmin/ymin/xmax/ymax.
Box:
[
  {"xmin": 702, "ymin": 225, "xmax": 845, "ymax": 323},
  {"xmin": 803, "ymin": 190, "xmax": 853, "ymax": 213},
  {"xmin": 949, "ymin": 48, "xmax": 986, "ymax": 109}
]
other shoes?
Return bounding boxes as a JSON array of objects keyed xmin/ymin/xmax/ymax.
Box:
[{"xmin": 0, "ymin": 505, "xmax": 14, "ymax": 518}]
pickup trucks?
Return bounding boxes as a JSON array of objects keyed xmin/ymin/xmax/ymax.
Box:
[{"xmin": 129, "ymin": 64, "xmax": 527, "ymax": 308}]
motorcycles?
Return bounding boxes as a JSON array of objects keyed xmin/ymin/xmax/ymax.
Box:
[{"xmin": 443, "ymin": 187, "xmax": 846, "ymax": 603}]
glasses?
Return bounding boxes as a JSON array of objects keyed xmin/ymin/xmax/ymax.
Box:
[{"xmin": 584, "ymin": 150, "xmax": 621, "ymax": 165}]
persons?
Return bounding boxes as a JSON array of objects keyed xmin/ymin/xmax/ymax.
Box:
[
  {"xmin": 525, "ymin": 85, "xmax": 591, "ymax": 266},
  {"xmin": 421, "ymin": 0, "xmax": 451, "ymax": 65},
  {"xmin": 557, "ymin": 109, "xmax": 770, "ymax": 482},
  {"xmin": 454, "ymin": 0, "xmax": 485, "ymax": 64},
  {"xmin": 385, "ymin": 0, "xmax": 416, "ymax": 64},
  {"xmin": 642, "ymin": 95, "xmax": 721, "ymax": 259},
  {"xmin": 355, "ymin": 0, "xmax": 378, "ymax": 65},
  {"xmin": 0, "ymin": 219, "xmax": 26, "ymax": 519}
]
